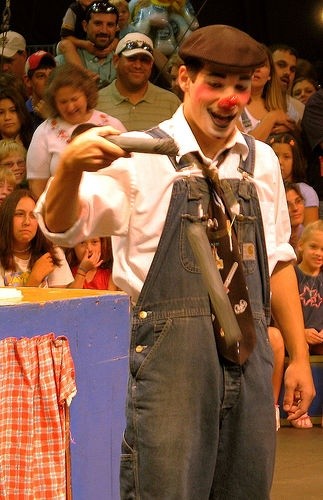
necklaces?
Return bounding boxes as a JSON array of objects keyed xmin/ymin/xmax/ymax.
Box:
[{"xmin": 12, "ymin": 244, "xmax": 32, "ymax": 253}]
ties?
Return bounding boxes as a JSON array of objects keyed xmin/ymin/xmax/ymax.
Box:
[{"xmin": 191, "ymin": 147, "xmax": 257, "ymax": 366}]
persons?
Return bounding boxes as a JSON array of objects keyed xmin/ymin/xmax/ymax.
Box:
[
  {"xmin": 0, "ymin": 0, "xmax": 323, "ymax": 429},
  {"xmin": 34, "ymin": 23, "xmax": 318, "ymax": 500}
]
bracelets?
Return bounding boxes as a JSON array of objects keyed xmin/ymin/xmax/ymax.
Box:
[{"xmin": 76, "ymin": 272, "xmax": 86, "ymax": 277}]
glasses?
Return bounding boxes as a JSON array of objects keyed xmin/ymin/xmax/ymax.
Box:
[
  {"xmin": 87, "ymin": 2, "xmax": 116, "ymax": 13},
  {"xmin": 117, "ymin": 41, "xmax": 153, "ymax": 55},
  {"xmin": 1, "ymin": 159, "xmax": 26, "ymax": 168}
]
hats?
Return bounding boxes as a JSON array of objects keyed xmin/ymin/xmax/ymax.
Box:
[
  {"xmin": 178, "ymin": 24, "xmax": 267, "ymax": 71},
  {"xmin": 0, "ymin": 31, "xmax": 25, "ymax": 57},
  {"xmin": 25, "ymin": 50, "xmax": 56, "ymax": 75},
  {"xmin": 114, "ymin": 33, "xmax": 155, "ymax": 62}
]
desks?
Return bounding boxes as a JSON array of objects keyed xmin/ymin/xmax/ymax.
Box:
[{"xmin": 0, "ymin": 286, "xmax": 132, "ymax": 500}]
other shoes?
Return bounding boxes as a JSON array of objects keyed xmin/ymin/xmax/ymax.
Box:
[{"xmin": 288, "ymin": 390, "xmax": 313, "ymax": 429}]
